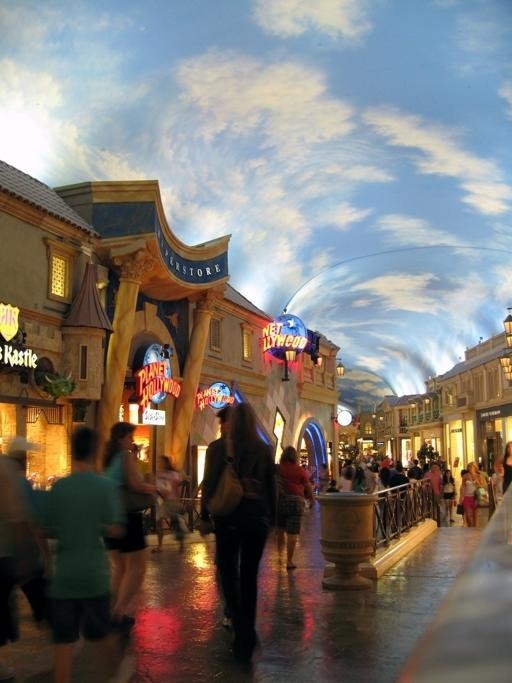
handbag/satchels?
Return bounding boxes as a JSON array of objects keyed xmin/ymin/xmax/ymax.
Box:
[
  {"xmin": 477, "ymin": 486, "xmax": 488, "ymax": 505},
  {"xmin": 117, "ymin": 454, "xmax": 158, "ymax": 513},
  {"xmin": 457, "ymin": 503, "xmax": 464, "ymax": 513},
  {"xmin": 206, "ymin": 436, "xmax": 242, "ymax": 515},
  {"xmin": 272, "ymin": 476, "xmax": 286, "ymax": 514}
]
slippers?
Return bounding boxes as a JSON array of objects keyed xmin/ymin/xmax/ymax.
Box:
[{"xmin": 286, "ymin": 563, "xmax": 296, "ymax": 570}]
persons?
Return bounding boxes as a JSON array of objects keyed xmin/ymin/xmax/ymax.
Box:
[
  {"xmin": 215, "ymin": 399, "xmax": 278, "ymax": 664},
  {"xmin": 100, "ymin": 419, "xmax": 158, "ymax": 628},
  {"xmin": 192, "ymin": 400, "xmax": 235, "ymax": 631},
  {"xmin": 150, "ymin": 451, "xmax": 193, "ymax": 556},
  {"xmin": 7, "ymin": 435, "xmax": 53, "ymax": 529},
  {"xmin": 27, "ymin": 425, "xmax": 127, "ymax": 679},
  {"xmin": 0, "ymin": 452, "xmax": 51, "ymax": 646},
  {"xmin": 318, "ymin": 441, "xmax": 511, "ymax": 527},
  {"xmin": 272, "ymin": 443, "xmax": 316, "ymax": 571},
  {"xmin": 130, "ymin": 443, "xmax": 150, "ymax": 517}
]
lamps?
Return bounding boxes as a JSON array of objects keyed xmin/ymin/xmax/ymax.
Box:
[
  {"xmin": 263, "ymin": 341, "xmax": 345, "ymax": 376},
  {"xmin": 498, "ymin": 306, "xmax": 511, "ymax": 387}
]
